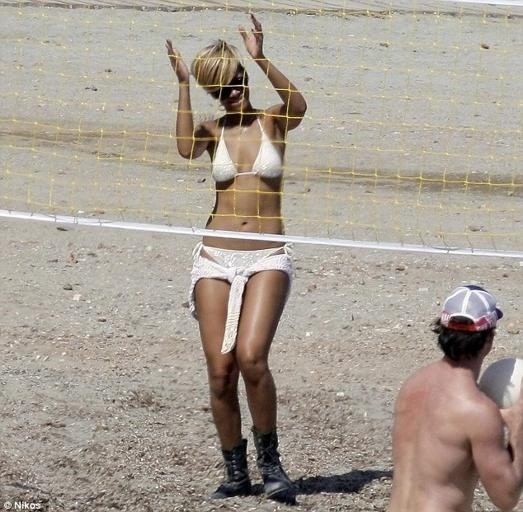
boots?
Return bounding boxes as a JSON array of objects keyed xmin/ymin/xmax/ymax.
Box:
[
  {"xmin": 251, "ymin": 426, "xmax": 291, "ymax": 499},
  {"xmin": 211, "ymin": 438, "xmax": 251, "ymax": 499}
]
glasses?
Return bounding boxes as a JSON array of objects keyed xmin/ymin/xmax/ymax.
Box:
[{"xmin": 212, "ymin": 77, "xmax": 247, "ymax": 100}]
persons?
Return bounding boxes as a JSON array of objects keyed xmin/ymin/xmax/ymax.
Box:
[
  {"xmin": 165, "ymin": 13, "xmax": 306, "ymax": 499},
  {"xmin": 387, "ymin": 285, "xmax": 523, "ymax": 512}
]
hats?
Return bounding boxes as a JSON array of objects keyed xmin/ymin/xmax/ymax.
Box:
[{"xmin": 441, "ymin": 283, "xmax": 503, "ymax": 332}]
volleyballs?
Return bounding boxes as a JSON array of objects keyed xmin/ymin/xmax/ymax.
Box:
[{"xmin": 479, "ymin": 358, "xmax": 522, "ymax": 448}]
